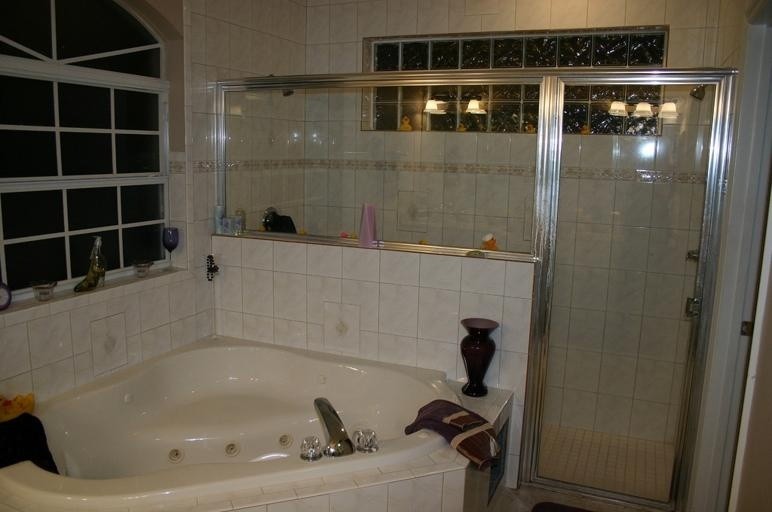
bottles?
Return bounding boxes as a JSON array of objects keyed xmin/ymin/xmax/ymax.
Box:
[{"xmin": 215, "ymin": 204, "xmax": 245, "ymax": 236}]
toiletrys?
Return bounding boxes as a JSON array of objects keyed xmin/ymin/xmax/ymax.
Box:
[{"xmin": 214, "ymin": 202, "xmax": 247, "ymax": 236}]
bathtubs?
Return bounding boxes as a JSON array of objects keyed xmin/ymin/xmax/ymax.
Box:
[{"xmin": 0, "ymin": 333, "xmax": 514, "ymax": 512}]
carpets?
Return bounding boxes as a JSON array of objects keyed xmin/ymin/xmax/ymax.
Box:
[{"xmin": 531, "ymin": 502, "xmax": 591, "ymax": 512}]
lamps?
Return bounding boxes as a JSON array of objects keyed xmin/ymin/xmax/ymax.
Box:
[
  {"xmin": 423, "ymin": 99, "xmax": 447, "ymax": 115},
  {"xmin": 465, "ymin": 99, "xmax": 487, "ymax": 114},
  {"xmin": 608, "ymin": 101, "xmax": 679, "ymax": 119}
]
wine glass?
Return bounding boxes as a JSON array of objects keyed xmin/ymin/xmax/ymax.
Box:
[{"xmin": 162, "ymin": 227, "xmax": 180, "ymax": 273}]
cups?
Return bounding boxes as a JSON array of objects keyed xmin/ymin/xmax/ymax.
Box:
[
  {"xmin": 132, "ymin": 259, "xmax": 153, "ymax": 277},
  {"xmin": 31, "ymin": 280, "xmax": 57, "ymax": 303}
]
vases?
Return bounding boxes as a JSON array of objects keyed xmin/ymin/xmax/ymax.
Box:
[{"xmin": 460, "ymin": 318, "xmax": 499, "ymax": 397}]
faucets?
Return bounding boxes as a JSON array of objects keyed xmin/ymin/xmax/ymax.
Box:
[{"xmin": 314, "ymin": 396, "xmax": 355, "ymax": 457}]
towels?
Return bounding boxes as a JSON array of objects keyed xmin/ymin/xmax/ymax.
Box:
[{"xmin": 404, "ymin": 399, "xmax": 500, "ymax": 471}]
